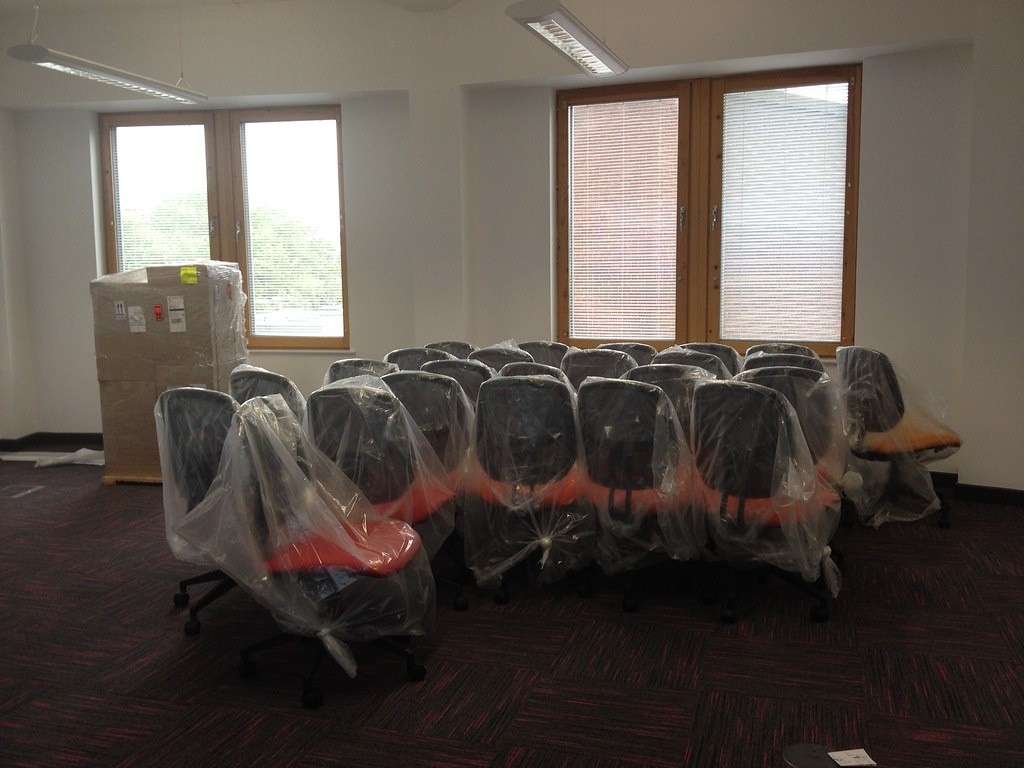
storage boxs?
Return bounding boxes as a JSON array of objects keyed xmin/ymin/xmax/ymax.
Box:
[{"xmin": 91, "ymin": 262, "xmax": 250, "ymax": 488}]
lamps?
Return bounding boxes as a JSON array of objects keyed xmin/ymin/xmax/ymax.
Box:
[
  {"xmin": 6, "ymin": 0, "xmax": 209, "ymax": 106},
  {"xmin": 503, "ymin": 0, "xmax": 631, "ymax": 79}
]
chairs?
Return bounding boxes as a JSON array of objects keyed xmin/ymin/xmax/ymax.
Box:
[{"xmin": 156, "ymin": 339, "xmax": 963, "ymax": 707}]
